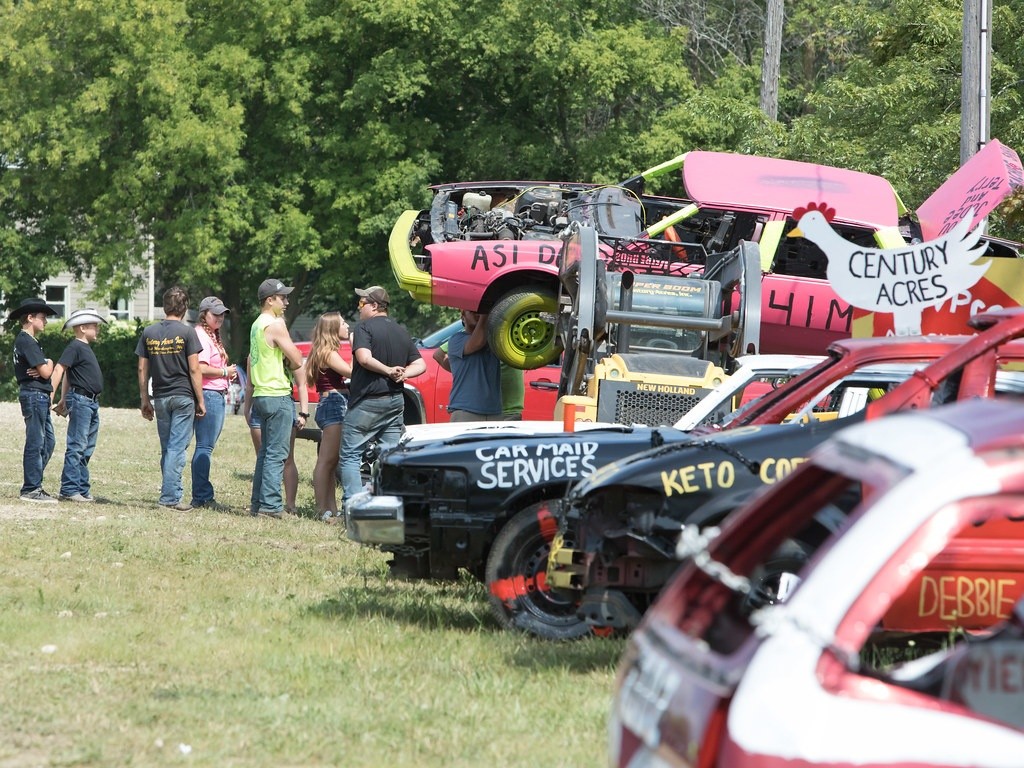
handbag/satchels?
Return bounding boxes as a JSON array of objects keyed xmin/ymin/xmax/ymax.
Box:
[{"xmin": 224, "ymin": 374, "xmax": 244, "ymax": 406}]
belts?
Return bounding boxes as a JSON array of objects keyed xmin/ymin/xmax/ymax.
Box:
[{"xmin": 74, "ymin": 388, "xmax": 100, "ymax": 403}]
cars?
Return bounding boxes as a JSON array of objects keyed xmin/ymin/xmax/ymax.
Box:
[{"xmin": 288, "ymin": 149, "xmax": 1024, "ymax": 768}]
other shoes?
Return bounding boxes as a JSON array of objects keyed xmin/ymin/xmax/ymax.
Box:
[
  {"xmin": 191, "ymin": 498, "xmax": 231, "ymax": 513},
  {"xmin": 320, "ymin": 511, "xmax": 342, "ymax": 524},
  {"xmin": 250, "ymin": 508, "xmax": 298, "ymax": 520}
]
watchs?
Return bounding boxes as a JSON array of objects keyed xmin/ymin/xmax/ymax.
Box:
[{"xmin": 298, "ymin": 411, "xmax": 310, "ymax": 418}]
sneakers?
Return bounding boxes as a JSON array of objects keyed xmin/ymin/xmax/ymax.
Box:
[
  {"xmin": 84, "ymin": 494, "xmax": 102, "ymax": 503},
  {"xmin": 58, "ymin": 493, "xmax": 93, "ymax": 502},
  {"xmin": 156, "ymin": 502, "xmax": 194, "ymax": 513},
  {"xmin": 20, "ymin": 487, "xmax": 58, "ymax": 504}
]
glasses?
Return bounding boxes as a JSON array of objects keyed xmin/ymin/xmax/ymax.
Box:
[{"xmin": 359, "ymin": 301, "xmax": 373, "ymax": 308}]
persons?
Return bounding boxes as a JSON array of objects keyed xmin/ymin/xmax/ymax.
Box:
[
  {"xmin": 50, "ymin": 308, "xmax": 108, "ymax": 502},
  {"xmin": 337, "ymin": 286, "xmax": 427, "ymax": 518},
  {"xmin": 8, "ymin": 297, "xmax": 59, "ymax": 503},
  {"xmin": 448, "ymin": 309, "xmax": 502, "ymax": 421},
  {"xmin": 304, "ymin": 311, "xmax": 354, "ymax": 521},
  {"xmin": 242, "ymin": 278, "xmax": 310, "ymax": 520},
  {"xmin": 133, "ymin": 285, "xmax": 238, "ymax": 513},
  {"xmin": 433, "ymin": 318, "xmax": 524, "ymax": 420}
]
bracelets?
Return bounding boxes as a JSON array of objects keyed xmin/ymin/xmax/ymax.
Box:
[{"xmin": 221, "ymin": 368, "xmax": 228, "ymax": 378}]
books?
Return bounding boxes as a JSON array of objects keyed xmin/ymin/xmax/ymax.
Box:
[{"xmin": 52, "ymin": 403, "xmax": 69, "ymax": 418}]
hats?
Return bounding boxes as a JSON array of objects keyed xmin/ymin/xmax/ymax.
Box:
[
  {"xmin": 258, "ymin": 279, "xmax": 295, "ymax": 301},
  {"xmin": 199, "ymin": 296, "xmax": 232, "ymax": 316},
  {"xmin": 355, "ymin": 286, "xmax": 390, "ymax": 304},
  {"xmin": 10, "ymin": 298, "xmax": 58, "ymax": 320},
  {"xmin": 61, "ymin": 308, "xmax": 109, "ymax": 332}
]
